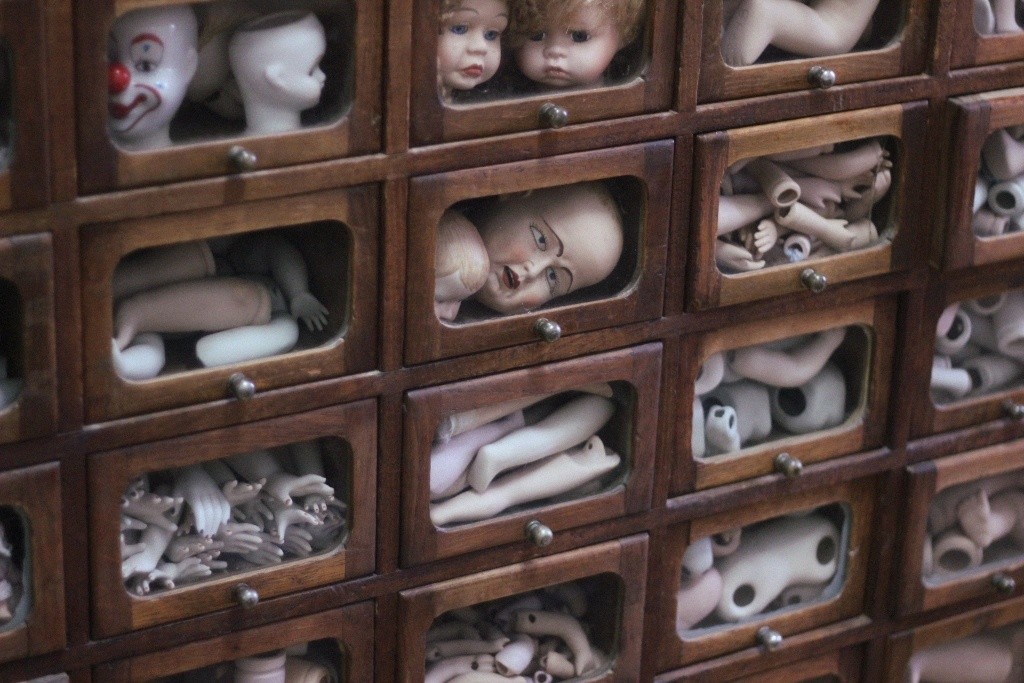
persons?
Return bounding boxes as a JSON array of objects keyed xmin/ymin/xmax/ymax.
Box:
[{"xmin": 0, "ymin": 0, "xmax": 1024, "ymax": 682}]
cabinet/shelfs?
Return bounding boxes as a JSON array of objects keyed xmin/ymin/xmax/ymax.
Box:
[{"xmin": 1, "ymin": 0, "xmax": 1021, "ymax": 683}]
